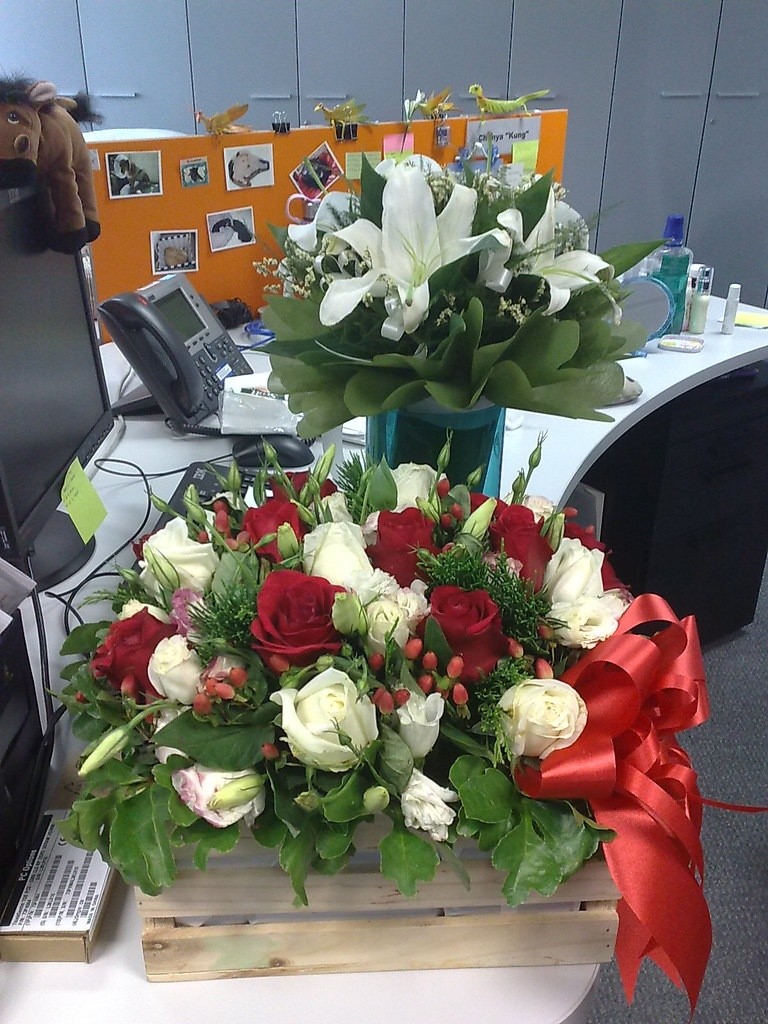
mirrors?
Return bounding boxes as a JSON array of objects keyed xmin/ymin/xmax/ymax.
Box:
[{"xmin": 619, "ymin": 275, "xmax": 676, "ymax": 356}]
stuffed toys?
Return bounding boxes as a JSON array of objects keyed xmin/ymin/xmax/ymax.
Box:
[{"xmin": 0, "ymin": 69, "xmax": 102, "ymax": 254}]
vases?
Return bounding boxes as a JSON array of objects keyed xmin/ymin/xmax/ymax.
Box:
[
  {"xmin": 136, "ymin": 800, "xmax": 623, "ymax": 984},
  {"xmin": 364, "ymin": 395, "xmax": 507, "ymax": 499}
]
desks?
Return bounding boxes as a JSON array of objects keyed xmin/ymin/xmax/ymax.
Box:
[
  {"xmin": 0, "ymin": 797, "xmax": 619, "ymax": 1023},
  {"xmin": 21, "ymin": 273, "xmax": 767, "ymax": 816}
]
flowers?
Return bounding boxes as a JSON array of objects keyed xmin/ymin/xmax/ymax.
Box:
[
  {"xmin": 47, "ymin": 435, "xmax": 618, "ymax": 908},
  {"xmin": 263, "ymin": 131, "xmax": 650, "ymax": 442}
]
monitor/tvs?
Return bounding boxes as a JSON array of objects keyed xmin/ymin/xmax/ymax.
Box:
[{"xmin": 0, "ymin": 183, "xmax": 115, "ymax": 594}]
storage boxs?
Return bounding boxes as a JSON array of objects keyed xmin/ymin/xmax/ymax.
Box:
[{"xmin": 0, "ymin": 746, "xmax": 135, "ymax": 963}]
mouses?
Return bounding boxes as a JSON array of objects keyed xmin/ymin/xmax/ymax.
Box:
[{"xmin": 232, "ymin": 434, "xmax": 315, "ymax": 468}]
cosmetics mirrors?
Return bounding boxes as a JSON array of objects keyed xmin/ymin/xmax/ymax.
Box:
[{"xmin": 619, "ymin": 275, "xmax": 674, "ymax": 357}]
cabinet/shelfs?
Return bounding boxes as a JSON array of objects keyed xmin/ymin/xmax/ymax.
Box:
[
  {"xmin": 0, "ymin": 0, "xmax": 767, "ymax": 311},
  {"xmin": 588, "ymin": 360, "xmax": 767, "ymax": 645}
]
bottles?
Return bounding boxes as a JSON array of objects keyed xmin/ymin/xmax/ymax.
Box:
[
  {"xmin": 720, "ymin": 284, "xmax": 742, "ymax": 334},
  {"xmin": 684, "ymin": 261, "xmax": 714, "ymax": 335},
  {"xmin": 644, "ymin": 213, "xmax": 694, "ymax": 338}
]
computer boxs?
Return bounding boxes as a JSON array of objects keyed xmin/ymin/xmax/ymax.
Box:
[{"xmin": 0, "ymin": 607, "xmax": 43, "ymax": 916}]
